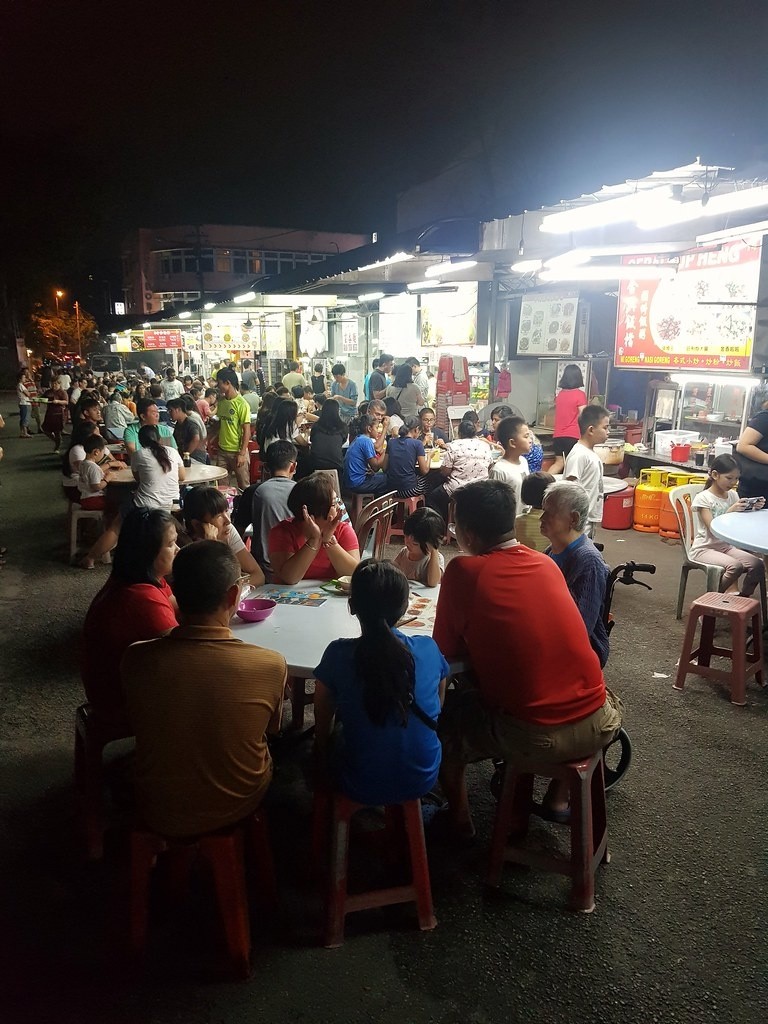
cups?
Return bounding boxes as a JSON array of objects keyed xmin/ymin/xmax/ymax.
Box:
[
  {"xmin": 715, "ymin": 444, "xmax": 732, "ymax": 458},
  {"xmin": 426, "ymin": 432, "xmax": 435, "ymax": 445},
  {"xmin": 696, "ymin": 454, "xmax": 704, "ymax": 466},
  {"xmin": 709, "ymin": 455, "xmax": 715, "ymax": 467},
  {"xmin": 628, "ymin": 410, "xmax": 638, "ymax": 422}
]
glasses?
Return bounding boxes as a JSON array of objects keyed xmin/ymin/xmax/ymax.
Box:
[{"xmin": 423, "ymin": 419, "xmax": 434, "ymax": 423}]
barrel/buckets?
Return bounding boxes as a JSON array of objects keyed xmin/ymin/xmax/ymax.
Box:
[
  {"xmin": 671, "ymin": 444, "xmax": 691, "ymax": 462},
  {"xmin": 602, "ymin": 486, "xmax": 634, "ymax": 530}
]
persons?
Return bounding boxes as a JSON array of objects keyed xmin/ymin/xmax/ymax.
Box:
[
  {"xmin": 60, "ymin": 396, "xmax": 212, "ymax": 568},
  {"xmin": 513, "ymin": 474, "xmax": 557, "ymax": 553},
  {"xmin": 254, "ymin": 360, "xmax": 360, "ymax": 485},
  {"xmin": 534, "ymin": 479, "xmax": 613, "ymax": 822},
  {"xmin": 16, "ymin": 367, "xmax": 43, "ymax": 437},
  {"xmin": 99, "ymin": 360, "xmax": 170, "ymax": 422},
  {"xmin": 343, "ymin": 396, "xmax": 544, "ymax": 545},
  {"xmin": 80, "ymin": 472, "xmax": 448, "ymax": 731},
  {"xmin": 124, "ymin": 541, "xmax": 292, "ymax": 930},
  {"xmin": 31, "ymin": 359, "xmax": 101, "ymax": 454},
  {"xmin": 312, "ymin": 558, "xmax": 452, "ymax": 805},
  {"xmin": 488, "ymin": 416, "xmax": 533, "ymax": 516},
  {"xmin": 689, "ymin": 400, "xmax": 768, "ymax": 638},
  {"xmin": 158, "ymin": 359, "xmax": 263, "ymax": 425},
  {"xmin": 213, "ymin": 369, "xmax": 252, "ymax": 492},
  {"xmin": 562, "ymin": 405, "xmax": 611, "ymax": 540},
  {"xmin": 430, "ymin": 478, "xmax": 620, "ymax": 847},
  {"xmin": 363, "ymin": 352, "xmax": 429, "ymax": 421},
  {"xmin": 547, "ymin": 364, "xmax": 587, "ymax": 474}
]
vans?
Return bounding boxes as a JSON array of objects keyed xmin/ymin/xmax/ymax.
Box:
[{"xmin": 86, "ymin": 352, "xmax": 125, "ymax": 381}]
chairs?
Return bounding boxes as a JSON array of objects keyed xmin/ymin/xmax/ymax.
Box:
[
  {"xmin": 352, "ymin": 490, "xmax": 399, "ymax": 561},
  {"xmin": 670, "ymin": 484, "xmax": 766, "ymax": 631}
]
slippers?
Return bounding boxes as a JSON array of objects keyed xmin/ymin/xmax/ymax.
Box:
[
  {"xmin": 543, "ymin": 803, "xmax": 570, "ymax": 816},
  {"xmin": 421, "ymin": 803, "xmax": 468, "ymax": 848},
  {"xmin": 78, "ymin": 560, "xmax": 95, "ymax": 570}
]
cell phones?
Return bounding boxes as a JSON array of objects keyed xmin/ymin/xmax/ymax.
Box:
[{"xmin": 744, "ymin": 497, "xmax": 761, "ymax": 507}]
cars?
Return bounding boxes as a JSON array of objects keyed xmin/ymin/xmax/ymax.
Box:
[{"xmin": 44, "ymin": 351, "xmax": 79, "ymax": 362}]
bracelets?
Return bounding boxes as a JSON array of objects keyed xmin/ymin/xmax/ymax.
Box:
[{"xmin": 305, "ymin": 542, "xmax": 316, "ymax": 551}]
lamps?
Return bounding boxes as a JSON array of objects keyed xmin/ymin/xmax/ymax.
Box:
[{"xmin": 509, "ymin": 185, "xmax": 768, "ymax": 283}]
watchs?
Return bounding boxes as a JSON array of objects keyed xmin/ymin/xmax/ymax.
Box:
[{"xmin": 322, "ymin": 535, "xmax": 338, "ymax": 549}]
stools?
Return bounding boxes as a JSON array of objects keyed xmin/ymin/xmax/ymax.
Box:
[
  {"xmin": 68, "ymin": 703, "xmax": 139, "ymax": 823},
  {"xmin": 127, "ymin": 828, "xmax": 259, "ymax": 972},
  {"xmin": 671, "ymin": 591, "xmax": 768, "ymax": 706},
  {"xmin": 436, "ymin": 356, "xmax": 469, "ymax": 437},
  {"xmin": 484, "ymin": 750, "xmax": 613, "ymax": 912},
  {"xmin": 384, "ymin": 494, "xmax": 426, "ymax": 545},
  {"xmin": 250, "ymin": 449, "xmax": 268, "ymax": 484},
  {"xmin": 351, "ymin": 493, "xmax": 374, "ymax": 519},
  {"xmin": 305, "ymin": 782, "xmax": 441, "ymax": 950},
  {"xmin": 70, "ymin": 508, "xmax": 112, "ymax": 566}
]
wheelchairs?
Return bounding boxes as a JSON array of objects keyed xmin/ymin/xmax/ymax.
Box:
[{"xmin": 491, "ymin": 541, "xmax": 657, "ymax": 825}]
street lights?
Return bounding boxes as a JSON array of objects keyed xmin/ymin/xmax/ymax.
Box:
[{"xmin": 56, "ymin": 291, "xmax": 63, "ymax": 319}]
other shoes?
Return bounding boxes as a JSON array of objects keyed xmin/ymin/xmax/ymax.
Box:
[
  {"xmin": 54, "ymin": 449, "xmax": 59, "ymax": 453},
  {"xmin": 38, "ymin": 431, "xmax": 43, "ymax": 433},
  {"xmin": 27, "ymin": 430, "xmax": 34, "ymax": 434},
  {"xmin": 20, "ymin": 434, "xmax": 32, "ymax": 438}
]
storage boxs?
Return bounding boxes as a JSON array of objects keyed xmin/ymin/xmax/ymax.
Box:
[{"xmin": 655, "ymin": 429, "xmax": 700, "ymax": 457}]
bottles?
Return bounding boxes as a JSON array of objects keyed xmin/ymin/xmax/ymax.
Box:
[{"xmin": 183, "ymin": 452, "xmax": 192, "ymax": 467}]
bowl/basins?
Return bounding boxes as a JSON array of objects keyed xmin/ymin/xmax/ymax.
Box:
[
  {"xmin": 32, "ymin": 398, "xmax": 48, "ymax": 402},
  {"xmin": 339, "ymin": 576, "xmax": 354, "ymax": 589},
  {"xmin": 236, "ymin": 599, "xmax": 277, "ymax": 622}
]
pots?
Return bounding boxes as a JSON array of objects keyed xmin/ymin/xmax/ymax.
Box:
[{"xmin": 592, "ymin": 438, "xmax": 626, "ymax": 465}]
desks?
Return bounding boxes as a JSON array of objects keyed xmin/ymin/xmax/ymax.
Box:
[
  {"xmin": 625, "ymin": 449, "xmax": 707, "ymax": 478},
  {"xmin": 549, "ymin": 473, "xmax": 629, "ymax": 512},
  {"xmin": 709, "ymin": 508, "xmax": 768, "ymax": 556},
  {"xmin": 104, "ymin": 466, "xmax": 228, "ymax": 511},
  {"xmin": 236, "ymin": 573, "xmax": 469, "ymax": 742}
]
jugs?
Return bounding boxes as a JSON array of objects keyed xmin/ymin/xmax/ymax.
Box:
[{"xmin": 607, "ymin": 404, "xmax": 622, "ymax": 420}]
